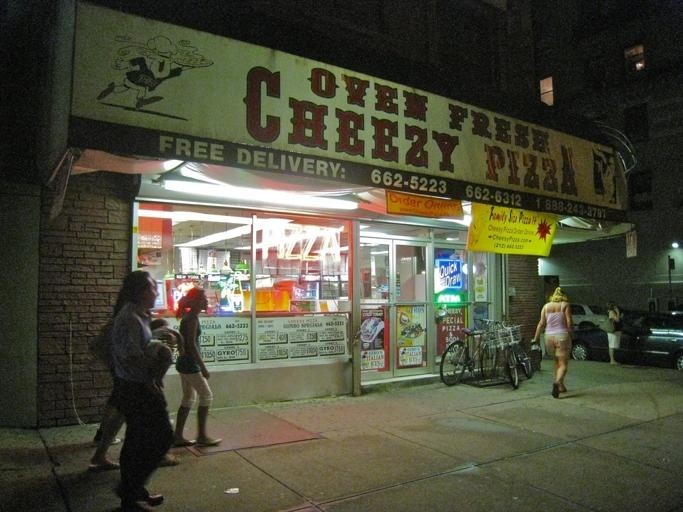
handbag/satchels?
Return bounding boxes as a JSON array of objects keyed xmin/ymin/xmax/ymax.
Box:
[{"xmin": 600, "ymin": 318, "xmax": 618, "ymax": 333}]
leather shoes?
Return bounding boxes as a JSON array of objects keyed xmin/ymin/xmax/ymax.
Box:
[
  {"xmin": 197, "ymin": 438, "xmax": 223, "ymax": 448},
  {"xmin": 146, "ymin": 493, "xmax": 165, "ymax": 506},
  {"xmin": 131, "ymin": 504, "xmax": 154, "ymax": 512},
  {"xmin": 175, "ymin": 439, "xmax": 197, "ymax": 446}
]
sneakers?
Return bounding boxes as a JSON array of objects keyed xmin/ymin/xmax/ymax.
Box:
[{"xmin": 92, "ymin": 438, "xmax": 121, "ymax": 445}]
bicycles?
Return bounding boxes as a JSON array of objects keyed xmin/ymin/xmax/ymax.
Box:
[
  {"xmin": 436, "ymin": 318, "xmax": 501, "ymax": 387},
  {"xmin": 493, "ymin": 320, "xmax": 532, "ymax": 388}
]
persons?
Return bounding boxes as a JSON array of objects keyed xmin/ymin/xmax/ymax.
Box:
[
  {"xmin": 533, "ymin": 287, "xmax": 575, "ymax": 397},
  {"xmin": 88, "ymin": 270, "xmax": 223, "ymax": 512},
  {"xmin": 606, "ymin": 299, "xmax": 624, "ymax": 366}
]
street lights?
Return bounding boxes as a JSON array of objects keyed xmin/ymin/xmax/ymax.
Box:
[{"xmin": 666, "ymin": 254, "xmax": 677, "ymax": 310}]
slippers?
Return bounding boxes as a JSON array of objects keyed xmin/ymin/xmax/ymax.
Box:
[
  {"xmin": 552, "ymin": 381, "xmax": 559, "ymax": 398},
  {"xmin": 90, "ymin": 458, "xmax": 120, "ymax": 472},
  {"xmin": 158, "ymin": 453, "xmax": 178, "ymax": 467}
]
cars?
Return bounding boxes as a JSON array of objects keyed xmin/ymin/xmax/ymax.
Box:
[
  {"xmin": 569, "ymin": 312, "xmax": 683, "ymax": 370},
  {"xmin": 567, "ymin": 302, "xmax": 608, "ymax": 329}
]
